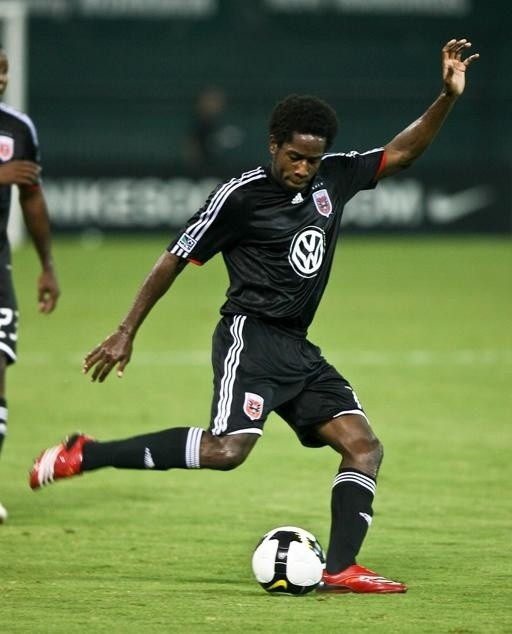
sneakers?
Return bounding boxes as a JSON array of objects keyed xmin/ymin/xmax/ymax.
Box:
[
  {"xmin": 316, "ymin": 564, "xmax": 408, "ymax": 593},
  {"xmin": 30, "ymin": 432, "xmax": 95, "ymax": 490}
]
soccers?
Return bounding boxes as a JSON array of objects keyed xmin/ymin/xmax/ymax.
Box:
[{"xmin": 251, "ymin": 527, "xmax": 327, "ymax": 596}]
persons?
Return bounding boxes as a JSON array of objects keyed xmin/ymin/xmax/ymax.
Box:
[
  {"xmin": 176, "ymin": 87, "xmax": 230, "ymax": 180},
  {"xmin": 1, "ymin": 46, "xmax": 62, "ymax": 529},
  {"xmin": 26, "ymin": 32, "xmax": 486, "ymax": 595}
]
